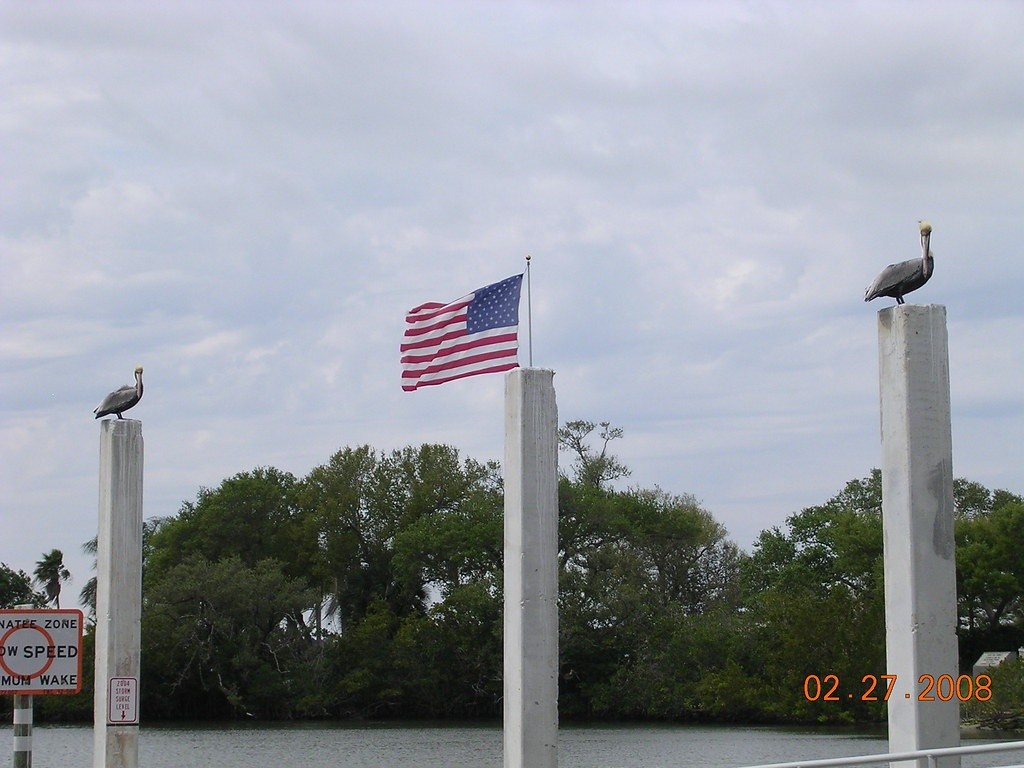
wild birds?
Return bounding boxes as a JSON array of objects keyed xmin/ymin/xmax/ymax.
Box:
[
  {"xmin": 864, "ymin": 219, "xmax": 935, "ymax": 305},
  {"xmin": 93, "ymin": 365, "xmax": 143, "ymax": 419}
]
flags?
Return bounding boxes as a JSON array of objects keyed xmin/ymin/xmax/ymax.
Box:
[{"xmin": 400, "ymin": 274, "xmax": 525, "ymax": 392}]
